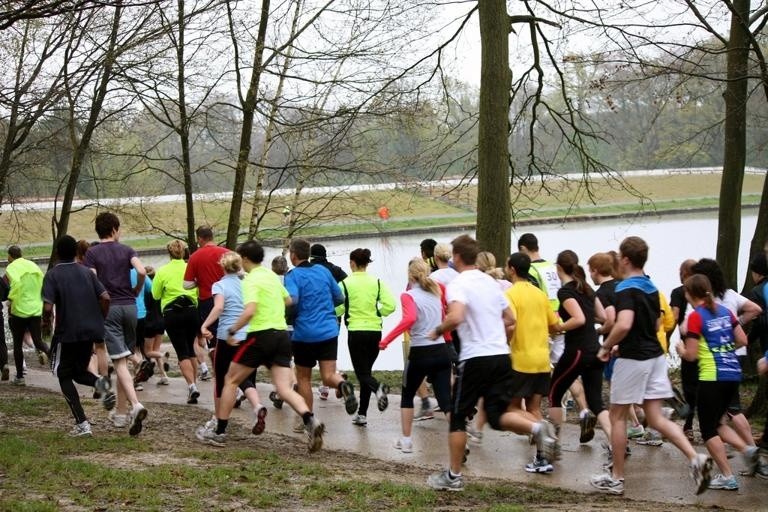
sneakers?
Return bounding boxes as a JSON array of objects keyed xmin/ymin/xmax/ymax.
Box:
[
  {"xmin": 392, "ymin": 437, "xmax": 415, "ymax": 452},
  {"xmin": 1, "ymin": 363, "xmax": 11, "ymax": 382},
  {"xmin": 132, "ymin": 351, "xmax": 172, "ymax": 392},
  {"xmin": 127, "ymin": 402, "xmax": 146, "ymax": 437},
  {"xmin": 8, "ymin": 366, "xmax": 28, "ymax": 387},
  {"xmin": 525, "ymin": 399, "xmax": 573, "ymax": 473},
  {"xmin": 690, "ymin": 442, "xmax": 768, "ymax": 496},
  {"xmin": 93, "ymin": 363, "xmax": 116, "ymax": 409},
  {"xmin": 106, "ymin": 408, "xmax": 127, "ymax": 427},
  {"xmin": 188, "ymin": 370, "xmax": 284, "ymax": 448},
  {"xmin": 291, "ymin": 373, "xmax": 390, "ymax": 452},
  {"xmin": 578, "ymin": 405, "xmax": 694, "ymax": 498},
  {"xmin": 413, "ymin": 399, "xmax": 443, "ymax": 421},
  {"xmin": 67, "ymin": 420, "xmax": 93, "ymax": 437},
  {"xmin": 426, "ymin": 415, "xmax": 487, "ymax": 493}
]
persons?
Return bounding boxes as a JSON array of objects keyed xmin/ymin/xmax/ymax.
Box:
[{"xmin": 1, "ymin": 212, "xmax": 767, "ymax": 494}]
